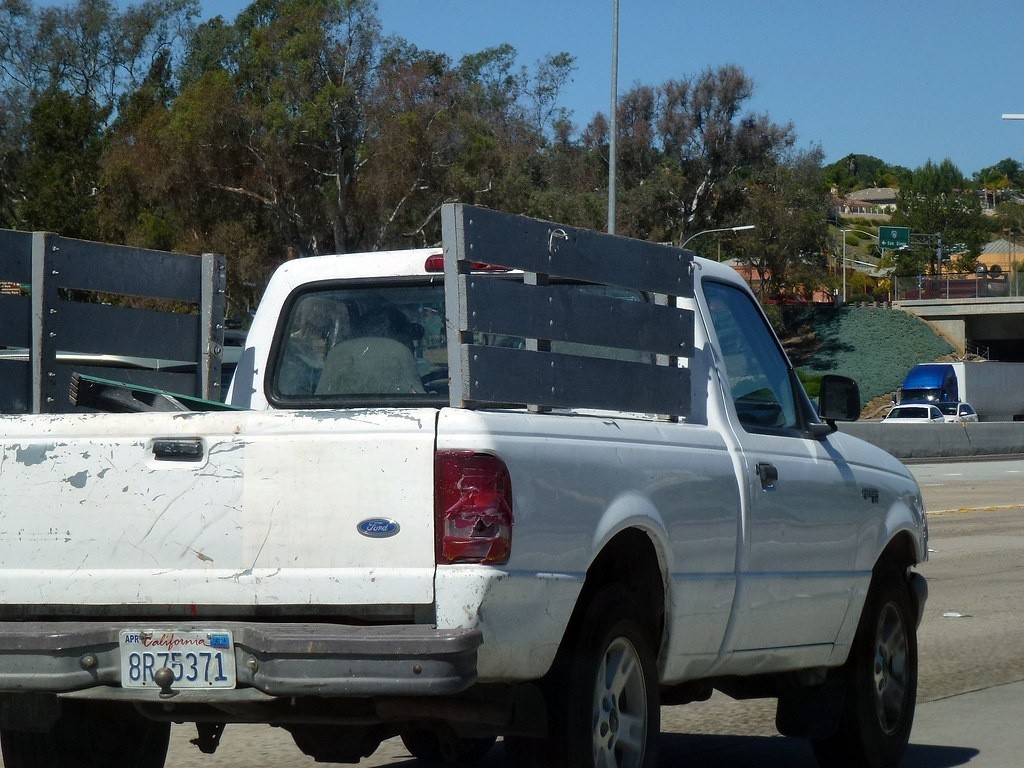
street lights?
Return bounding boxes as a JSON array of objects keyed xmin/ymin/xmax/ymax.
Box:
[
  {"xmin": 822, "ymin": 219, "xmax": 846, "ymax": 305},
  {"xmin": 680, "ymin": 224, "xmax": 755, "ymax": 249}
]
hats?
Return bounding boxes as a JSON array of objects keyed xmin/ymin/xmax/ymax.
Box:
[{"xmin": 348, "ymin": 305, "xmax": 425, "ymax": 343}]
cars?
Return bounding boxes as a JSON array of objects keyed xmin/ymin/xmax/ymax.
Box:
[
  {"xmin": 933, "ymin": 402, "xmax": 978, "ymax": 422},
  {"xmin": 881, "ymin": 404, "xmax": 946, "ymax": 423}
]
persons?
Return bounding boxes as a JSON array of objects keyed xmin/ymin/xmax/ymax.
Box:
[{"xmin": 351, "ymin": 305, "xmax": 438, "ymax": 394}]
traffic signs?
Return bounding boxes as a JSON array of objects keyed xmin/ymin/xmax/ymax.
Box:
[{"xmin": 878, "ymin": 226, "xmax": 910, "ymax": 248}]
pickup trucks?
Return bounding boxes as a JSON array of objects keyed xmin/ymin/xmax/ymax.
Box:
[{"xmin": 0, "ymin": 230, "xmax": 928, "ymax": 767}]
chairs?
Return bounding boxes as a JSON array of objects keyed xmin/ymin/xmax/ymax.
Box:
[{"xmin": 314, "ymin": 337, "xmax": 425, "ymax": 394}]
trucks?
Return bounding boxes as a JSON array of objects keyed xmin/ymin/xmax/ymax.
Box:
[{"xmin": 891, "ymin": 361, "xmax": 1024, "ymax": 421}]
